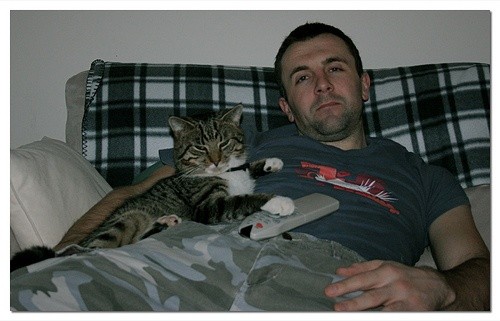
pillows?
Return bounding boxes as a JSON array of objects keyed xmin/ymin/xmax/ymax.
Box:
[{"xmin": 10, "ymin": 139, "xmax": 114, "ymax": 252}]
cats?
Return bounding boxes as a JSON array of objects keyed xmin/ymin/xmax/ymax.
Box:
[{"xmin": 10, "ymin": 102, "xmax": 295, "ymax": 272}]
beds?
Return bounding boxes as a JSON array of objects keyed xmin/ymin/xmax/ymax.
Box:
[{"xmin": 65, "ymin": 61, "xmax": 491, "ymax": 271}]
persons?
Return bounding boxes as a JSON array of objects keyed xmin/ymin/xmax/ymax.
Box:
[{"xmin": 10, "ymin": 21, "xmax": 490, "ymax": 312}]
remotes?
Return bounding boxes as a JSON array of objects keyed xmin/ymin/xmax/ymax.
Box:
[{"xmin": 239, "ymin": 193, "xmax": 340, "ymax": 241}]
